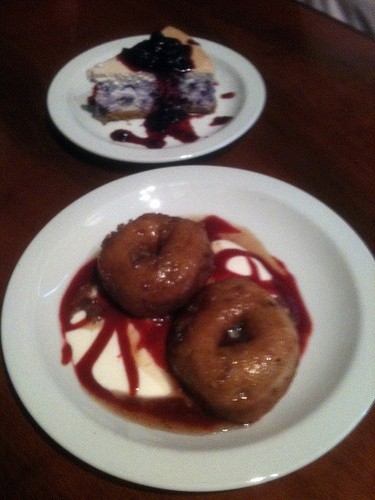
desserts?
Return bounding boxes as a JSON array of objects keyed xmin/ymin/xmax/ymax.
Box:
[
  {"xmin": 95, "ymin": 210, "xmax": 301, "ymax": 423},
  {"xmin": 85, "ymin": 26, "xmax": 219, "ymax": 125}
]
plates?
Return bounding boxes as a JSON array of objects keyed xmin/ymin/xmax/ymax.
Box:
[
  {"xmin": 1, "ymin": 164, "xmax": 375, "ymax": 493},
  {"xmin": 46, "ymin": 35, "xmax": 268, "ymax": 164}
]
